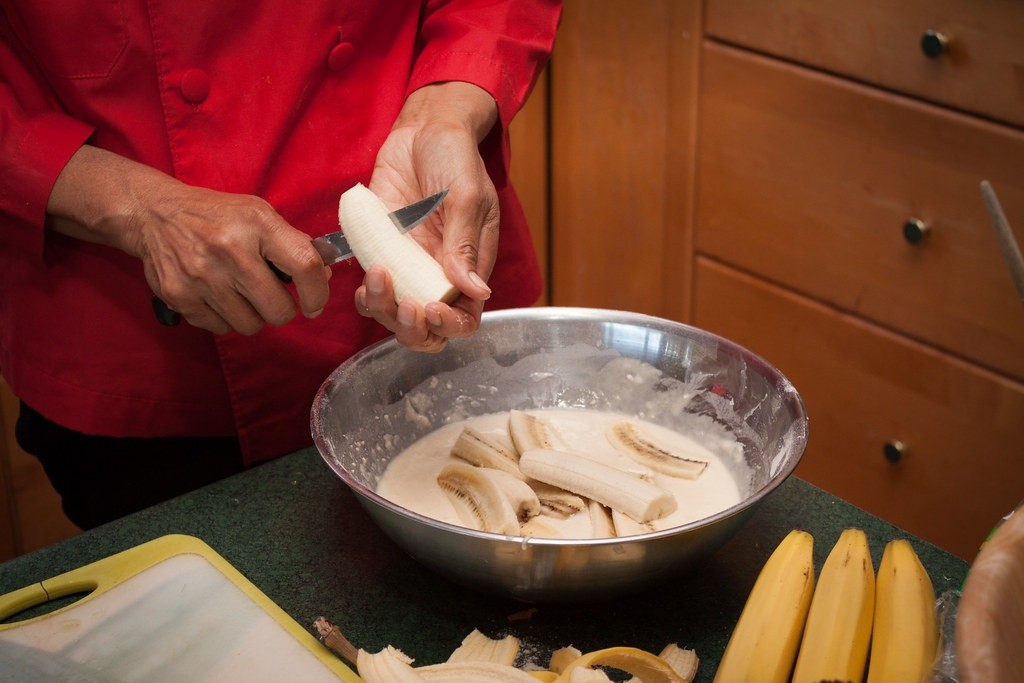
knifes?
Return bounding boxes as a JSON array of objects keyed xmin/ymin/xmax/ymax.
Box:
[{"xmin": 153, "ymin": 188, "xmax": 451, "ymax": 326}]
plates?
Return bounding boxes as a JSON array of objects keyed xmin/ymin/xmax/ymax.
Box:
[{"xmin": 0, "ymin": 532, "xmax": 365, "ymax": 682}]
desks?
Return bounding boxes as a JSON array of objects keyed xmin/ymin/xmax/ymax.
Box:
[{"xmin": 0, "ymin": 445, "xmax": 968, "ymax": 683}]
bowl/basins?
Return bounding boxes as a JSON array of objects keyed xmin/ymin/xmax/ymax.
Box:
[
  {"xmin": 311, "ymin": 305, "xmax": 813, "ymax": 606},
  {"xmin": 953, "ymin": 502, "xmax": 1024, "ymax": 683}
]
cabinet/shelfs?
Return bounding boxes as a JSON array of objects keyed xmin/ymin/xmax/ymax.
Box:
[{"xmin": 542, "ymin": 0, "xmax": 1024, "ymax": 564}]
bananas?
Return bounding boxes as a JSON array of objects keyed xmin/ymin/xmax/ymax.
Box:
[
  {"xmin": 713, "ymin": 527, "xmax": 937, "ymax": 683},
  {"xmin": 436, "ymin": 408, "xmax": 708, "ymax": 540},
  {"xmin": 339, "ymin": 184, "xmax": 459, "ymax": 308}
]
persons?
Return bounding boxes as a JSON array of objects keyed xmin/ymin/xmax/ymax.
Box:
[{"xmin": 0, "ymin": 0, "xmax": 566, "ymax": 532}]
